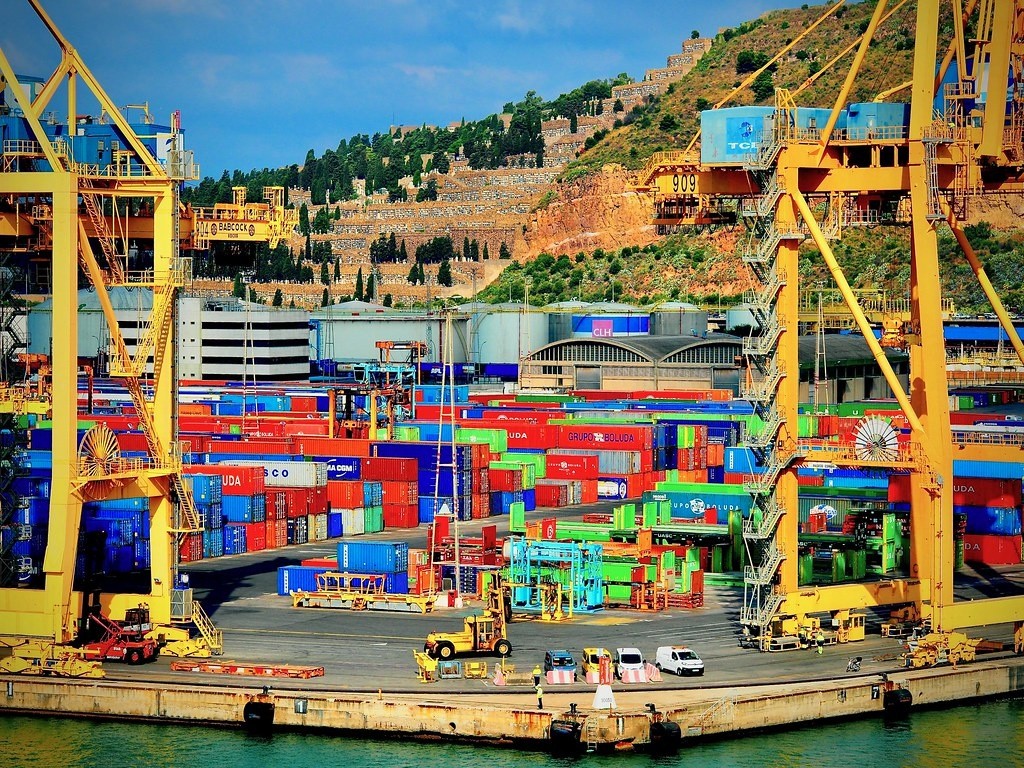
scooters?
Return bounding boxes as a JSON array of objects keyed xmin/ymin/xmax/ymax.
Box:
[{"xmin": 845, "ymin": 656, "xmax": 862, "ymax": 673}]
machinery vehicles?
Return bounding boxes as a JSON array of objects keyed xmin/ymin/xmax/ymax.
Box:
[
  {"xmin": 423, "ymin": 569, "xmax": 512, "ymax": 661},
  {"xmin": 84, "ymin": 604, "xmax": 161, "ymax": 665}
]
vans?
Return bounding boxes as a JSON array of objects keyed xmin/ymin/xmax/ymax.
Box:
[
  {"xmin": 615, "ymin": 647, "xmax": 646, "ymax": 680},
  {"xmin": 581, "ymin": 647, "xmax": 615, "ymax": 681},
  {"xmin": 544, "ymin": 650, "xmax": 578, "ymax": 682},
  {"xmin": 655, "ymin": 646, "xmax": 704, "ymax": 676}
]
